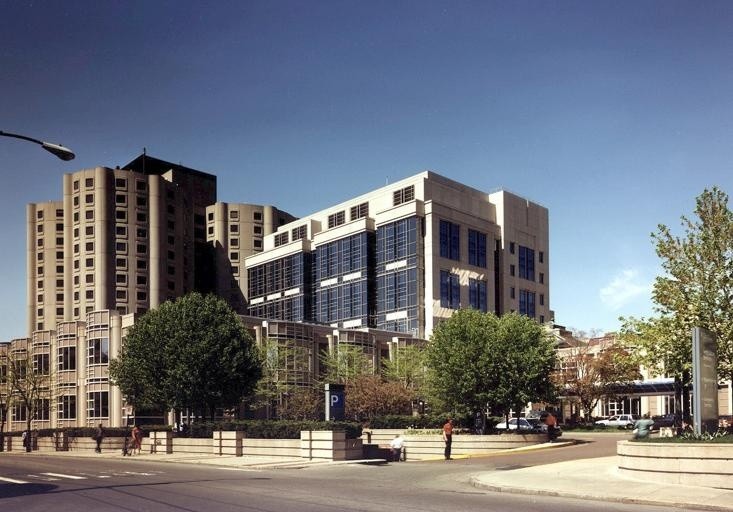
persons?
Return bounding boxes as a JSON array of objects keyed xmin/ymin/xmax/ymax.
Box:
[
  {"xmin": 94, "ymin": 423, "xmax": 105, "ymax": 453},
  {"xmin": 131, "ymin": 423, "xmax": 143, "ymax": 455},
  {"xmin": 633, "ymin": 413, "xmax": 656, "ymax": 438},
  {"xmin": 389, "ymin": 433, "xmax": 404, "ymax": 461},
  {"xmin": 544, "ymin": 410, "xmax": 559, "ymax": 442},
  {"xmin": 21, "ymin": 429, "xmax": 26, "ymax": 451},
  {"xmin": 442, "ymin": 418, "xmax": 455, "ymax": 460}
]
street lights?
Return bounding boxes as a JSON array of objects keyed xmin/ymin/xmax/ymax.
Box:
[{"xmin": 0, "ymin": 131, "xmax": 75, "ymax": 160}]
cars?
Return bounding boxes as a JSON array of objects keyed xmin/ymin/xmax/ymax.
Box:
[
  {"xmin": 595, "ymin": 414, "xmax": 680, "ymax": 430},
  {"xmin": 495, "ymin": 417, "xmax": 562, "ymax": 440}
]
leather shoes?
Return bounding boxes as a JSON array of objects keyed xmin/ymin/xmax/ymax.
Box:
[{"xmin": 445, "ymin": 459, "xmax": 453, "ymax": 460}]
